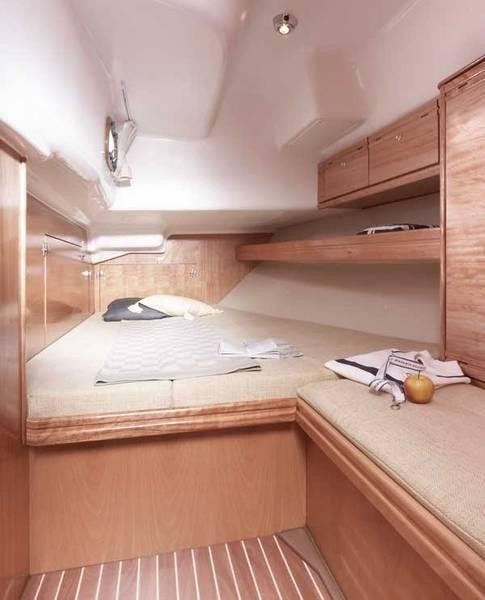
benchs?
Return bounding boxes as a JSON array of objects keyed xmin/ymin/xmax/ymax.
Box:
[{"xmin": 295, "ymin": 379, "xmax": 483, "ymax": 600}]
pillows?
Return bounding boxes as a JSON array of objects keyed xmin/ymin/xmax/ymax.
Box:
[{"xmin": 101, "ymin": 293, "xmax": 222, "ymax": 323}]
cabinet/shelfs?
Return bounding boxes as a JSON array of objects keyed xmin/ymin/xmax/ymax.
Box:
[
  {"xmin": 438, "ymin": 58, "xmax": 483, "ymax": 387},
  {"xmin": 315, "ymin": 97, "xmax": 439, "ymax": 211}
]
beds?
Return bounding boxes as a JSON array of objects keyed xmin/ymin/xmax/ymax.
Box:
[{"xmin": 26, "ymin": 195, "xmax": 443, "ymax": 575}]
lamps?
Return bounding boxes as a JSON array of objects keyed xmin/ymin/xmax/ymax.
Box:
[{"xmin": 272, "ymin": 14, "xmax": 297, "ymax": 35}]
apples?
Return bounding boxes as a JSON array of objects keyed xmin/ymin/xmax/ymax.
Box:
[{"xmin": 403, "ymin": 370, "xmax": 435, "ymax": 405}]
